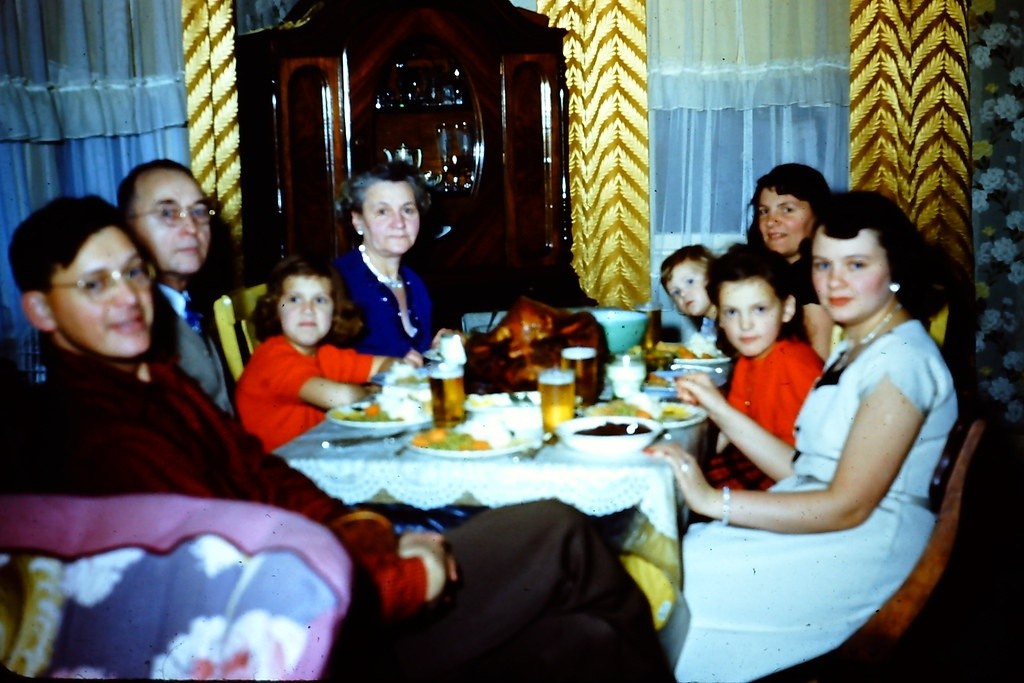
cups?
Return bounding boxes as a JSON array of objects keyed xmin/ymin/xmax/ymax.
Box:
[
  {"xmin": 605, "ymin": 354, "xmax": 646, "ymax": 398},
  {"xmin": 560, "ymin": 347, "xmax": 597, "ymax": 408},
  {"xmin": 426, "ymin": 362, "xmax": 465, "ymax": 428},
  {"xmin": 469, "ymin": 324, "xmax": 495, "ymax": 334},
  {"xmin": 538, "ymin": 368, "xmax": 575, "ymax": 437}
]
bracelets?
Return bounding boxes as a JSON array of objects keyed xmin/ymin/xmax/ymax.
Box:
[{"xmin": 722, "ymin": 486, "xmax": 730, "ymax": 527}]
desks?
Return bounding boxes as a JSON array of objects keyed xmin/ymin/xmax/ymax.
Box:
[{"xmin": 270, "ymin": 343, "xmax": 709, "ymax": 630}]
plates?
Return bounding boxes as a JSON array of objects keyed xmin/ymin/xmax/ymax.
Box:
[
  {"xmin": 324, "ymin": 347, "xmax": 583, "ymax": 462},
  {"xmin": 582, "ymin": 357, "xmax": 731, "ymax": 429}
]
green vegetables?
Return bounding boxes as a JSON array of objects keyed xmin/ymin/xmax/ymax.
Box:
[
  {"xmin": 429, "ymin": 431, "xmax": 470, "ymax": 451},
  {"xmin": 343, "ymin": 410, "xmax": 403, "ymax": 421},
  {"xmin": 605, "ymin": 399, "xmax": 639, "ymax": 417}
]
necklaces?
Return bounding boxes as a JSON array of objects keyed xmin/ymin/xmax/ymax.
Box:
[{"xmin": 853, "ymin": 304, "xmax": 902, "ymax": 345}]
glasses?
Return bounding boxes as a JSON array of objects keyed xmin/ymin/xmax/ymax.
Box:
[
  {"xmin": 52, "ymin": 262, "xmax": 155, "ymax": 303},
  {"xmin": 128, "ymin": 203, "xmax": 218, "ymax": 228}
]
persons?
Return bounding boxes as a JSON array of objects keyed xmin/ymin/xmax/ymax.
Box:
[
  {"xmin": 235, "ymin": 258, "xmax": 424, "ymax": 454},
  {"xmin": 334, "ymin": 160, "xmax": 435, "ymax": 365},
  {"xmin": 116, "ymin": 160, "xmax": 235, "ymax": 422},
  {"xmin": 677, "ymin": 243, "xmax": 823, "ymax": 529},
  {"xmin": 657, "ymin": 189, "xmax": 958, "ymax": 683},
  {"xmin": 658, "ymin": 244, "xmax": 719, "ymax": 344},
  {"xmin": 744, "ymin": 164, "xmax": 846, "ymax": 363},
  {"xmin": 0, "ymin": 194, "xmax": 676, "ymax": 683}
]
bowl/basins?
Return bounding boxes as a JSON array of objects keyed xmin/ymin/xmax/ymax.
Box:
[{"xmin": 589, "ymin": 309, "xmax": 647, "ymax": 353}]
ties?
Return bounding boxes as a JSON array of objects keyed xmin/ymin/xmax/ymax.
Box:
[{"xmin": 184, "ymin": 303, "xmax": 204, "ymax": 338}]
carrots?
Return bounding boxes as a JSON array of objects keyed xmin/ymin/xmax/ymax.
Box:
[
  {"xmin": 636, "ymin": 410, "xmax": 650, "ymax": 419},
  {"xmin": 646, "ymin": 373, "xmax": 665, "ymax": 382},
  {"xmin": 678, "ymin": 346, "xmax": 713, "ymax": 359},
  {"xmin": 413, "ymin": 428, "xmax": 447, "ymax": 446},
  {"xmin": 471, "ymin": 440, "xmax": 489, "ymax": 450},
  {"xmin": 366, "ymin": 403, "xmax": 379, "ymax": 416}
]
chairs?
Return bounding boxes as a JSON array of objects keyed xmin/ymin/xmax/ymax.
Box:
[
  {"xmin": 755, "ymin": 414, "xmax": 986, "ymax": 683},
  {"xmin": 214, "ymin": 285, "xmax": 268, "ymax": 382}
]
